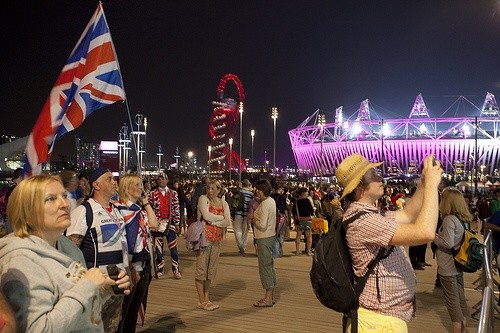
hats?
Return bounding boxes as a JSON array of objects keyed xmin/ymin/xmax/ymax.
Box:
[
  {"xmin": 156, "ymin": 172, "xmax": 168, "ymax": 181},
  {"xmin": 89, "ymin": 167, "xmax": 111, "ymax": 187},
  {"xmin": 335, "ymin": 155, "xmax": 385, "ymax": 200}
]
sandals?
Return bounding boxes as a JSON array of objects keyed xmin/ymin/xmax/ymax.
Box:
[{"xmin": 199, "ymin": 301, "xmax": 220, "ymax": 311}]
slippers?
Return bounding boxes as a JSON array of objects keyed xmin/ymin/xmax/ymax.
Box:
[{"xmin": 253, "ymin": 298, "xmax": 275, "ymax": 306}]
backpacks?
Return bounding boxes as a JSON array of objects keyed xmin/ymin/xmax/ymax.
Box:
[
  {"xmin": 310, "ymin": 211, "xmax": 386, "ymax": 313},
  {"xmin": 231, "ymin": 191, "xmax": 244, "ymax": 210},
  {"xmin": 452, "ymin": 229, "xmax": 487, "ymax": 273}
]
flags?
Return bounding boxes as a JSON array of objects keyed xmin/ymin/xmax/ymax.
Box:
[{"xmin": 22, "ymin": 0, "xmax": 126, "ymax": 179}]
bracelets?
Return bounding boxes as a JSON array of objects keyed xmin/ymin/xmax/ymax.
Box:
[
  {"xmin": 143, "ymin": 203, "xmax": 150, "ymax": 206},
  {"xmin": 251, "ymin": 218, "xmax": 254, "ymax": 221}
]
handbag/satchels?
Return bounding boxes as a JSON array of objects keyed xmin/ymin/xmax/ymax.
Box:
[
  {"xmin": 150, "ymin": 218, "xmax": 171, "ymax": 236},
  {"xmin": 183, "ymin": 221, "xmax": 208, "ymax": 251}
]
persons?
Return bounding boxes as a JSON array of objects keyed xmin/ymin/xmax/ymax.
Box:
[
  {"xmin": 433, "ymin": 186, "xmax": 474, "ymax": 333},
  {"xmin": 195, "ymin": 180, "xmax": 230, "ymax": 311},
  {"xmin": 149, "ymin": 172, "xmax": 182, "ymax": 280},
  {"xmin": 374, "ymin": 177, "xmax": 500, "ymax": 270},
  {"xmin": 66, "ymin": 167, "xmax": 141, "ymax": 333},
  {"xmin": 251, "ymin": 179, "xmax": 276, "ymax": 307},
  {"xmin": 169, "ymin": 176, "xmax": 344, "ymax": 256},
  {"xmin": 335, "ymin": 154, "xmax": 444, "ymax": 333},
  {"xmin": 0, "ymin": 174, "xmax": 131, "ymax": 333},
  {"xmin": 114, "ymin": 173, "xmax": 152, "ymax": 333},
  {"xmin": 0, "ymin": 168, "xmax": 91, "ymax": 224}
]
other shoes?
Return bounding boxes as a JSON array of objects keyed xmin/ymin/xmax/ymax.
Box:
[
  {"xmin": 412, "ymin": 262, "xmax": 432, "ymax": 270},
  {"xmin": 156, "ymin": 273, "xmax": 165, "ymax": 279},
  {"xmin": 307, "ymin": 251, "xmax": 314, "ymax": 256},
  {"xmin": 174, "ymin": 272, "xmax": 181, "ymax": 279}
]
